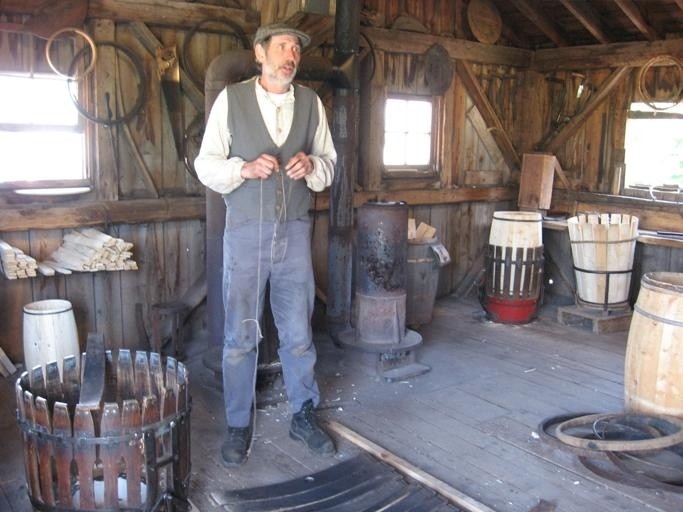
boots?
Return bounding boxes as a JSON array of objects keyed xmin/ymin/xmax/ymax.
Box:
[
  {"xmin": 288, "ymin": 399, "xmax": 336, "ymax": 458},
  {"xmin": 222, "ymin": 426, "xmax": 253, "ymax": 466}
]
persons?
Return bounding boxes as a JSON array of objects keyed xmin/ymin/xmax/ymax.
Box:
[{"xmin": 195, "ymin": 22, "xmax": 336, "ymax": 469}]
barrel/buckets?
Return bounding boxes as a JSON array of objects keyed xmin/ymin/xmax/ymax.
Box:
[
  {"xmin": 403, "ymin": 236, "xmax": 443, "ymax": 325},
  {"xmin": 21, "ymin": 299, "xmax": 81, "ymax": 385},
  {"xmin": 623, "ymin": 270, "xmax": 682, "ymax": 420},
  {"xmin": 485, "ymin": 209, "xmax": 545, "ymax": 297}
]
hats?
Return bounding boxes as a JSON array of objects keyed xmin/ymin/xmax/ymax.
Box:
[{"xmin": 254, "ymin": 24, "xmax": 311, "ymax": 50}]
